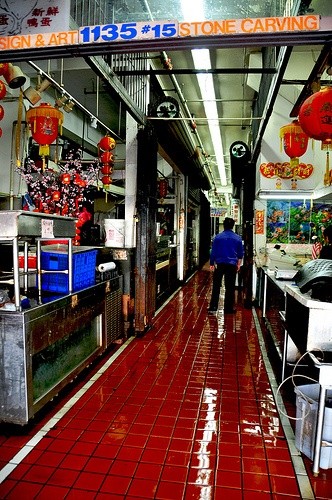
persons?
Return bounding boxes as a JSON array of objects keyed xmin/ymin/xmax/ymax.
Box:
[
  {"xmin": 207, "ymin": 218, "xmax": 244, "ymax": 313},
  {"xmin": 319, "ymin": 225, "xmax": 332, "ymax": 260}
]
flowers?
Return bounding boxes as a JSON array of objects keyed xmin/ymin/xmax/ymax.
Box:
[{"xmin": 9, "ymin": 146, "xmax": 103, "ymax": 217}]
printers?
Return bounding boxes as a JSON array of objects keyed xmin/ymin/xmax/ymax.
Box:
[{"xmin": 268, "ymin": 251, "xmax": 297, "ymax": 271}]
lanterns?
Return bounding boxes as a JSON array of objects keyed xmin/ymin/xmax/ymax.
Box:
[
  {"xmin": 100, "ymin": 134, "xmax": 116, "ymax": 188},
  {"xmin": 159, "ymin": 181, "xmax": 167, "ymax": 197},
  {"xmin": 298, "ymin": 86, "xmax": 332, "ymax": 152},
  {"xmin": 0, "ymin": 63, "xmax": 9, "ymax": 137},
  {"xmin": 26, "ymin": 103, "xmax": 64, "ymax": 156},
  {"xmin": 280, "ymin": 120, "xmax": 308, "ymax": 167}
]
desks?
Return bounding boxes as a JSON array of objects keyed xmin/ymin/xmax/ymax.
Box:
[{"xmin": 259, "ymin": 266, "xmax": 296, "ymax": 317}]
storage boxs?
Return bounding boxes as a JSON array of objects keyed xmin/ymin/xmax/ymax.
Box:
[
  {"xmin": 284, "ymin": 284, "xmax": 332, "ymax": 352},
  {"xmin": 294, "ymin": 383, "xmax": 332, "ymax": 470},
  {"xmin": 19, "ymin": 238, "xmax": 97, "ymax": 295}
]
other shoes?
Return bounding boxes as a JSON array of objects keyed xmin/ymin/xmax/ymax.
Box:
[
  {"xmin": 207, "ymin": 306, "xmax": 218, "ymax": 311},
  {"xmin": 224, "ymin": 309, "xmax": 236, "ymax": 314}
]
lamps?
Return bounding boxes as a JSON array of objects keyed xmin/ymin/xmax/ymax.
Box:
[
  {"xmin": 326, "ymin": 49, "xmax": 332, "ymax": 75},
  {"xmin": 90, "ymin": 116, "xmax": 98, "ymax": 128},
  {"xmin": 190, "ymin": 47, "xmax": 228, "ymax": 187},
  {"xmin": 23, "ymin": 71, "xmax": 52, "ymax": 106},
  {"xmin": 3, "ymin": 62, "xmax": 26, "ymax": 89},
  {"xmin": 55, "ymin": 91, "xmax": 76, "ymax": 113}
]
